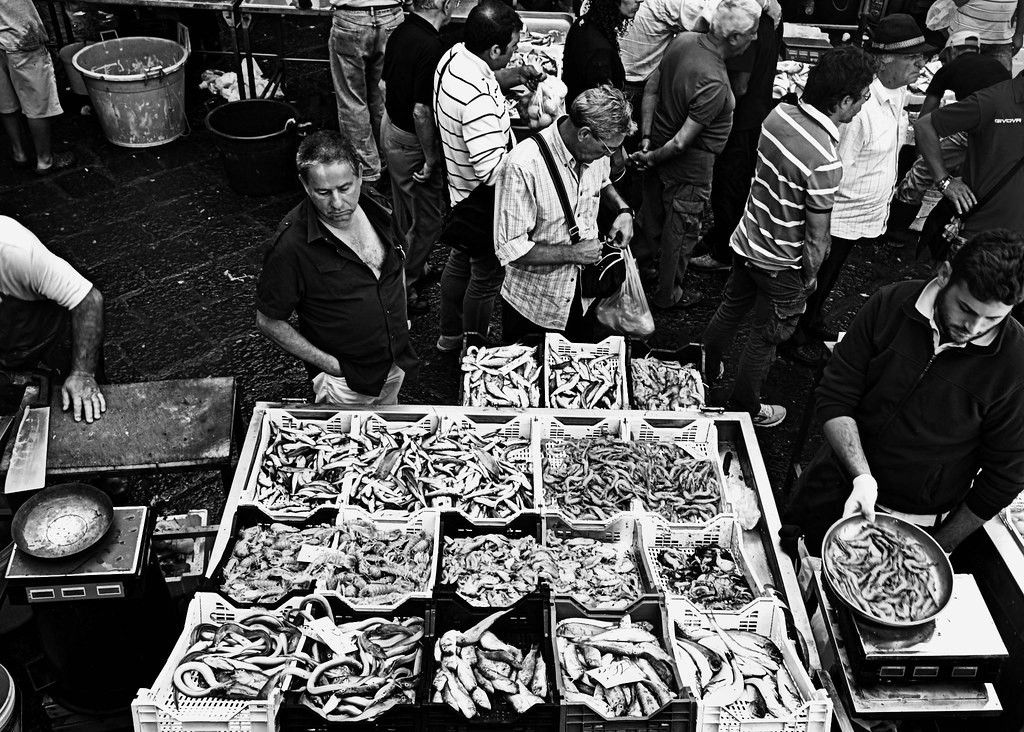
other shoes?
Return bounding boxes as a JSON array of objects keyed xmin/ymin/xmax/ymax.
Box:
[
  {"xmin": 652, "ymin": 289, "xmax": 705, "ymax": 309},
  {"xmin": 813, "ymin": 322, "xmax": 840, "ymax": 342},
  {"xmin": 422, "ymin": 263, "xmax": 445, "ymax": 281},
  {"xmin": 362, "ymin": 161, "xmax": 388, "ymax": 182},
  {"xmin": 14, "ymin": 155, "xmax": 39, "ymax": 170},
  {"xmin": 33, "ymin": 152, "xmax": 76, "ymax": 175},
  {"xmin": 407, "ymin": 295, "xmax": 430, "ymax": 314},
  {"xmin": 776, "ymin": 336, "xmax": 831, "ymax": 369}
]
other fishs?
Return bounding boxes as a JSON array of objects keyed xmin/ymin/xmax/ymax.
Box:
[
  {"xmin": 432, "ymin": 609, "xmax": 547, "ymax": 717},
  {"xmin": 350, "ymin": 416, "xmax": 534, "ymax": 516},
  {"xmin": 555, "ymin": 615, "xmax": 677, "ymax": 717},
  {"xmin": 171, "ymin": 595, "xmax": 423, "ymax": 722},
  {"xmin": 632, "ymin": 356, "xmax": 705, "ymax": 412},
  {"xmin": 442, "ymin": 534, "xmax": 638, "ymax": 609},
  {"xmin": 549, "ymin": 344, "xmax": 624, "ymax": 410},
  {"xmin": 256, "ymin": 418, "xmax": 356, "ymax": 510},
  {"xmin": 676, "ymin": 612, "xmax": 801, "ymax": 718},
  {"xmin": 462, "ymin": 344, "xmax": 542, "ymax": 408}
]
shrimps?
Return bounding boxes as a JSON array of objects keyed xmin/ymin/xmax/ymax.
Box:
[
  {"xmin": 541, "ymin": 433, "xmax": 718, "ymax": 522},
  {"xmin": 825, "ymin": 523, "xmax": 939, "ymax": 620},
  {"xmin": 220, "ymin": 523, "xmax": 331, "ymax": 601},
  {"xmin": 334, "ymin": 520, "xmax": 432, "ymax": 604}
]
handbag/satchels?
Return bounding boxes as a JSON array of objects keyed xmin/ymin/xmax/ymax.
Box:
[
  {"xmin": 577, "ymin": 239, "xmax": 626, "ymax": 299},
  {"xmin": 592, "ymin": 243, "xmax": 656, "ymax": 337},
  {"xmin": 914, "ymin": 197, "xmax": 958, "ymax": 254}
]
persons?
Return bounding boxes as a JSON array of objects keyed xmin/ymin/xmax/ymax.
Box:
[
  {"xmin": 289, "ymin": 0, "xmax": 416, "ymax": 181},
  {"xmin": 433, "ymin": 2, "xmax": 547, "ymax": 367},
  {"xmin": 913, "ymin": 69, "xmax": 1024, "ymax": 255},
  {"xmin": 377, "ymin": 0, "xmax": 461, "ymax": 287},
  {"xmin": 782, "ymin": 229, "xmax": 1024, "ymax": 555},
  {"xmin": 494, "ymin": 86, "xmax": 639, "ymax": 351},
  {"xmin": 776, "ymin": 14, "xmax": 934, "ymax": 364},
  {"xmin": 703, "ymin": 46, "xmax": 876, "ymax": 426},
  {"xmin": 563, "ymin": 0, "xmax": 783, "ymax": 305},
  {"xmin": 881, "ymin": 29, "xmax": 1012, "ymax": 252},
  {"xmin": 257, "ymin": 133, "xmax": 417, "ymax": 405},
  {"xmin": 0, "ymin": 213, "xmax": 106, "ymax": 423},
  {"xmin": 947, "ymin": 0, "xmax": 1024, "ymax": 69},
  {"xmin": 0, "ymin": 0, "xmax": 75, "ymax": 171}
]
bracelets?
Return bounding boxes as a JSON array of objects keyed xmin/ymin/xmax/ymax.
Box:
[
  {"xmin": 938, "ymin": 175, "xmax": 953, "ymax": 193},
  {"xmin": 643, "ymin": 135, "xmax": 650, "ymax": 139},
  {"xmin": 617, "ymin": 208, "xmax": 635, "ymax": 219},
  {"xmin": 935, "ymin": 175, "xmax": 949, "ymax": 186}
]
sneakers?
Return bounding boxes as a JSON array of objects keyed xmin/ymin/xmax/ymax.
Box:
[
  {"xmin": 436, "ymin": 333, "xmax": 465, "ymax": 352},
  {"xmin": 715, "ymin": 360, "xmax": 725, "ymax": 380},
  {"xmin": 751, "ymin": 403, "xmax": 787, "ymax": 428},
  {"xmin": 689, "ymin": 252, "xmax": 733, "ymax": 272}
]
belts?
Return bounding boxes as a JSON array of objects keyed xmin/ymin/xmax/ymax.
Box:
[
  {"xmin": 332, "ymin": 2, "xmax": 403, "ymax": 11},
  {"xmin": 874, "ymin": 501, "xmax": 951, "ymax": 527}
]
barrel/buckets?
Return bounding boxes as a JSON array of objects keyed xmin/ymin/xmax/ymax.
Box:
[
  {"xmin": 0, "ymin": 664, "xmax": 22, "ymax": 732},
  {"xmin": 72, "ymin": 37, "xmax": 189, "ymax": 148},
  {"xmin": 205, "ymin": 97, "xmax": 312, "ymax": 197},
  {"xmin": 60, "ymin": 41, "xmax": 95, "ymax": 96}
]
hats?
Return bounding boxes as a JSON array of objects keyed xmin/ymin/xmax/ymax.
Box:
[
  {"xmin": 863, "ymin": 13, "xmax": 939, "ymax": 54},
  {"xmin": 938, "ymin": 30, "xmax": 981, "ymax": 61}
]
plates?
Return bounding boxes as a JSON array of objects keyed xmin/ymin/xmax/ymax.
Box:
[
  {"xmin": 821, "ymin": 511, "xmax": 955, "ymax": 626},
  {"xmin": 11, "ymin": 483, "xmax": 114, "ymax": 559}
]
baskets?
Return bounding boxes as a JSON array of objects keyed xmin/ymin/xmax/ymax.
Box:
[{"xmin": 132, "ymin": 333, "xmax": 834, "ymax": 732}]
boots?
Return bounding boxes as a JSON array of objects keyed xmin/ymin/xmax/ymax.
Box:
[{"xmin": 881, "ymin": 193, "xmax": 923, "ymax": 249}]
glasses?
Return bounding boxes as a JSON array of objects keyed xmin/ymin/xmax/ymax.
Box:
[{"xmin": 596, "ymin": 132, "xmax": 618, "ymax": 158}]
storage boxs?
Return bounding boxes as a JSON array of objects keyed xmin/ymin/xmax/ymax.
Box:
[{"xmin": 130, "ymin": 332, "xmax": 833, "ymax": 732}]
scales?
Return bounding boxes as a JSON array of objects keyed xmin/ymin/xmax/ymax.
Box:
[
  {"xmin": 837, "ymin": 572, "xmax": 1010, "ymax": 688},
  {"xmin": 5, "ymin": 506, "xmax": 152, "ymax": 604}
]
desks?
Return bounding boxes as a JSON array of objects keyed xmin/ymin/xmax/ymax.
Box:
[
  {"xmin": 32, "ymin": 0, "xmax": 478, "ymax": 100},
  {"xmin": 0, "ymin": 377, "xmax": 244, "ymax": 515}
]
